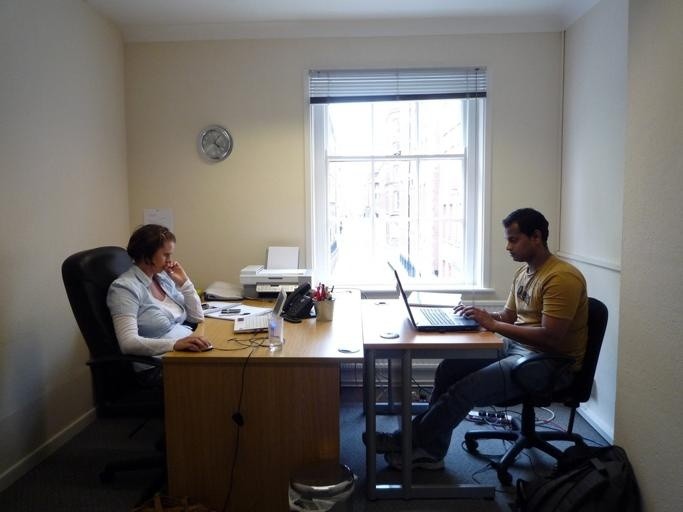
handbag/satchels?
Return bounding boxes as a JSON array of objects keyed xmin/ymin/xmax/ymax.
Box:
[{"xmin": 513, "ymin": 446, "xmax": 643, "ymax": 511}]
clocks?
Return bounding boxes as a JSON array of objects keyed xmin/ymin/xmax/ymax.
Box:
[{"xmin": 195, "ymin": 126, "xmax": 233, "ymax": 163}]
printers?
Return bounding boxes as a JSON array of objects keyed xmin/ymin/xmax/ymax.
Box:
[{"xmin": 239, "ymin": 265, "xmax": 312, "ymax": 300}]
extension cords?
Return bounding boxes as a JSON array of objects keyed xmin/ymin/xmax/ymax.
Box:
[{"xmin": 469, "ymin": 411, "xmax": 511, "ymax": 423}]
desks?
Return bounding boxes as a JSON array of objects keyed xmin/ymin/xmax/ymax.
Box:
[
  {"xmin": 162, "ymin": 287, "xmax": 364, "ymax": 512},
  {"xmin": 362, "ymin": 297, "xmax": 505, "ymax": 500}
]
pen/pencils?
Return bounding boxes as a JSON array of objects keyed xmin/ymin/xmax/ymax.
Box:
[
  {"xmin": 316, "ymin": 283, "xmax": 334, "ymax": 301},
  {"xmin": 221, "ymin": 308, "xmax": 241, "ymax": 314}
]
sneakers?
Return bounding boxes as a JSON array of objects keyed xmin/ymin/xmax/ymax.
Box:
[{"xmin": 363, "ymin": 430, "xmax": 445, "ymax": 471}]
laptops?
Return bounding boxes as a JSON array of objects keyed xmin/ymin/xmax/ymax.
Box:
[
  {"xmin": 234, "ymin": 287, "xmax": 287, "ymax": 333},
  {"xmin": 387, "ymin": 261, "xmax": 480, "ymax": 332}
]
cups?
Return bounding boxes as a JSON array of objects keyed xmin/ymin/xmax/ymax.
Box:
[
  {"xmin": 266, "ymin": 317, "xmax": 282, "ymax": 346},
  {"xmin": 312, "ymin": 297, "xmax": 335, "ymax": 320}
]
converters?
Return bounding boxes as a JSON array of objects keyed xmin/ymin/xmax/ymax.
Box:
[{"xmin": 231, "ymin": 412, "xmax": 243, "ymax": 426}]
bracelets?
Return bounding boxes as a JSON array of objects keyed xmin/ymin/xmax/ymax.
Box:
[
  {"xmin": 495, "ymin": 310, "xmax": 502, "ymax": 321},
  {"xmin": 492, "ymin": 321, "xmax": 498, "ymax": 333}
]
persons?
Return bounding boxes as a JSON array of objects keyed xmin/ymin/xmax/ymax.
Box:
[
  {"xmin": 106, "ymin": 224, "xmax": 210, "ymax": 451},
  {"xmin": 362, "ymin": 208, "xmax": 588, "ymax": 472}
]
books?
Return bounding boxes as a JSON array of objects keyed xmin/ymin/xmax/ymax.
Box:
[{"xmin": 200, "ymin": 280, "xmax": 272, "ymax": 321}]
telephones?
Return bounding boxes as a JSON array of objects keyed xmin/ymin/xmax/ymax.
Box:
[{"xmin": 284, "ymin": 282, "xmax": 314, "ymax": 318}]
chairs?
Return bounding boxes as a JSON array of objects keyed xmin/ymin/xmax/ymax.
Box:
[
  {"xmin": 464, "ymin": 296, "xmax": 610, "ymax": 485},
  {"xmin": 59, "ymin": 246, "xmax": 197, "ymax": 511}
]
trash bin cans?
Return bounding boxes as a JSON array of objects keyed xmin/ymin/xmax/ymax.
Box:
[{"xmin": 288, "ymin": 461, "xmax": 358, "ymax": 512}]
